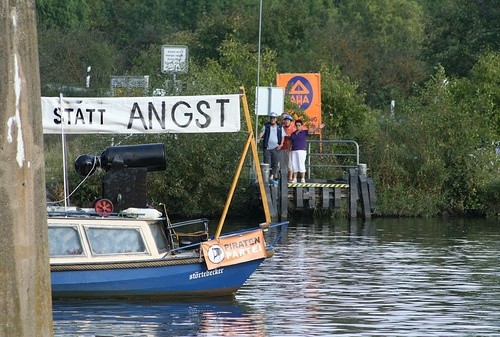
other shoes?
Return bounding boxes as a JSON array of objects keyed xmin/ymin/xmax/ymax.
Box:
[
  {"xmin": 289, "ymin": 178, "xmax": 297, "ymax": 183},
  {"xmin": 300, "ymin": 178, "xmax": 305, "ymax": 183}
]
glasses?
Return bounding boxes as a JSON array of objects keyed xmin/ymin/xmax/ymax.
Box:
[{"xmin": 270, "ymin": 116, "xmax": 276, "ymax": 119}]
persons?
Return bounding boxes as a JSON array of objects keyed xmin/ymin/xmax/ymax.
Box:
[
  {"xmin": 257, "ymin": 111, "xmax": 286, "ymax": 183},
  {"xmin": 280, "ymin": 116, "xmax": 296, "ymax": 184},
  {"xmin": 288, "ymin": 119, "xmax": 326, "ymax": 184},
  {"xmin": 279, "ymin": 112, "xmax": 288, "ymax": 126}
]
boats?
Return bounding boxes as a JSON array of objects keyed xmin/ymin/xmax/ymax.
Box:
[{"xmin": 44, "ymin": 143, "xmax": 292, "ymax": 299}]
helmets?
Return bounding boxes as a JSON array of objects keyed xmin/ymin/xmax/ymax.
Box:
[
  {"xmin": 284, "ymin": 115, "xmax": 293, "ymax": 121},
  {"xmin": 269, "ymin": 112, "xmax": 278, "ymax": 118}
]
